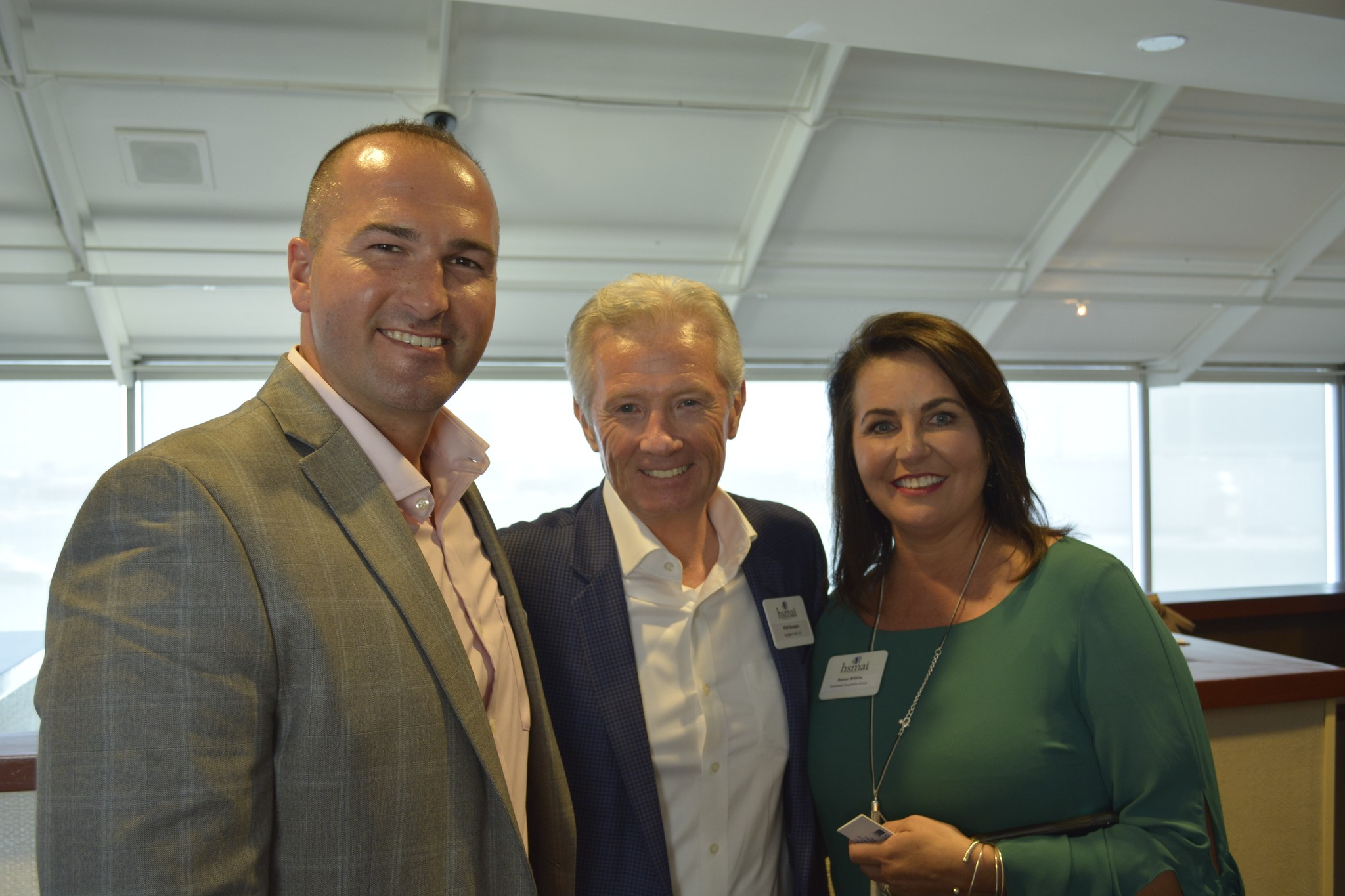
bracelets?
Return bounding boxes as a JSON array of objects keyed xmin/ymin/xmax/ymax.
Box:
[
  {"xmin": 952, "ymin": 839, "xmax": 981, "ymax": 896},
  {"xmin": 967, "ymin": 844, "xmax": 1005, "ymax": 896}
]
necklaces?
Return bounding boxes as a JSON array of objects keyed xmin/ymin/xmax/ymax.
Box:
[{"xmin": 869, "ymin": 523, "xmax": 992, "ymax": 896}]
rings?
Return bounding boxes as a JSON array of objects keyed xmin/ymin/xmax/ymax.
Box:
[{"xmin": 879, "ymin": 883, "xmax": 891, "ymax": 896}]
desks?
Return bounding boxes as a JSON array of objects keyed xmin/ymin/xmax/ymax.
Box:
[{"xmin": 1143, "ymin": 583, "xmax": 1345, "ymax": 896}]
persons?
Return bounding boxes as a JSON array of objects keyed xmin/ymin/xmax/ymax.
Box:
[
  {"xmin": 33, "ymin": 121, "xmax": 578, "ymax": 896},
  {"xmin": 804, "ymin": 311, "xmax": 1245, "ymax": 896},
  {"xmin": 495, "ymin": 276, "xmax": 833, "ymax": 895}
]
